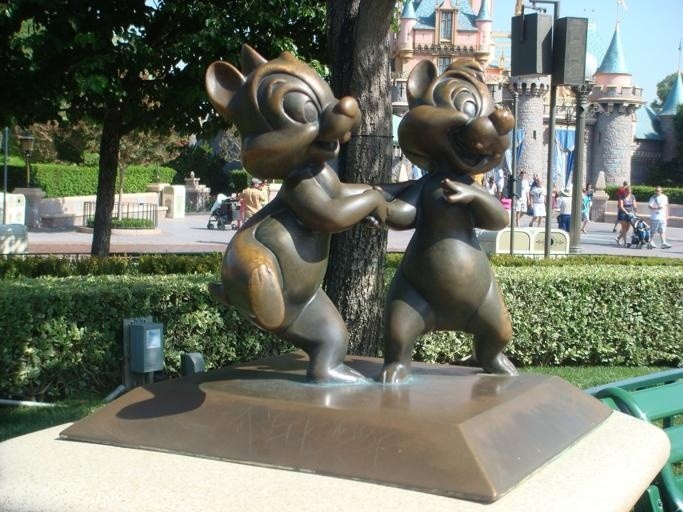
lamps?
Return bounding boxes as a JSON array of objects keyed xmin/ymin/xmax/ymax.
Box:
[{"xmin": 19, "ymin": 136, "xmax": 34, "ymax": 186}]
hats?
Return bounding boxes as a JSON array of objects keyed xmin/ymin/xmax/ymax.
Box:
[
  {"xmin": 560, "ymin": 184, "xmax": 572, "ymax": 196},
  {"xmin": 252, "ymin": 178, "xmax": 262, "ymax": 183}
]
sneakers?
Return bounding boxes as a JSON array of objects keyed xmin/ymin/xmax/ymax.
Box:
[
  {"xmin": 616, "ymin": 236, "xmax": 621, "ymax": 244},
  {"xmin": 613, "ymin": 228, "xmax": 618, "ymax": 232},
  {"xmin": 642, "ymin": 240, "xmax": 672, "ymax": 249},
  {"xmin": 581, "ymin": 227, "xmax": 587, "ymax": 233}
]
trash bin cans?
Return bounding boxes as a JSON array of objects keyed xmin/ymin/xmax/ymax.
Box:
[
  {"xmin": 0, "ymin": 194, "xmax": 25, "ymax": 224},
  {"xmin": 162, "ymin": 185, "xmax": 185, "ymax": 218}
]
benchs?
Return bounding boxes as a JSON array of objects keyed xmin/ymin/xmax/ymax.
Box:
[{"xmin": 595, "ymin": 380, "xmax": 683, "ymax": 512}]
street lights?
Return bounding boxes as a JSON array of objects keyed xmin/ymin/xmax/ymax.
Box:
[
  {"xmin": 18, "ymin": 128, "xmax": 37, "ymax": 189},
  {"xmin": 568, "ymin": 51, "xmax": 598, "ymax": 254}
]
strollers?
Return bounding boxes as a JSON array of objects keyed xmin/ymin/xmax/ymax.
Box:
[
  {"xmin": 627, "ymin": 216, "xmax": 653, "ymax": 250},
  {"xmin": 206, "ymin": 198, "xmax": 239, "ymax": 230}
]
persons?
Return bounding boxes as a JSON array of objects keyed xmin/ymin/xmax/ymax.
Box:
[
  {"xmin": 613, "ymin": 184, "xmax": 637, "ymax": 250},
  {"xmin": 645, "ymin": 184, "xmax": 673, "ymax": 250},
  {"xmin": 236, "ymin": 177, "xmax": 268, "ymax": 224},
  {"xmin": 484, "ymin": 176, "xmax": 497, "ymax": 196},
  {"xmin": 259, "ymin": 178, "xmax": 271, "ymax": 209},
  {"xmin": 635, "ymin": 219, "xmax": 651, "ymax": 244},
  {"xmin": 234, "ymin": 198, "xmax": 244, "ymax": 229},
  {"xmin": 513, "ymin": 170, "xmax": 593, "ymax": 234},
  {"xmin": 613, "ymin": 181, "xmax": 629, "ymax": 233}
]
